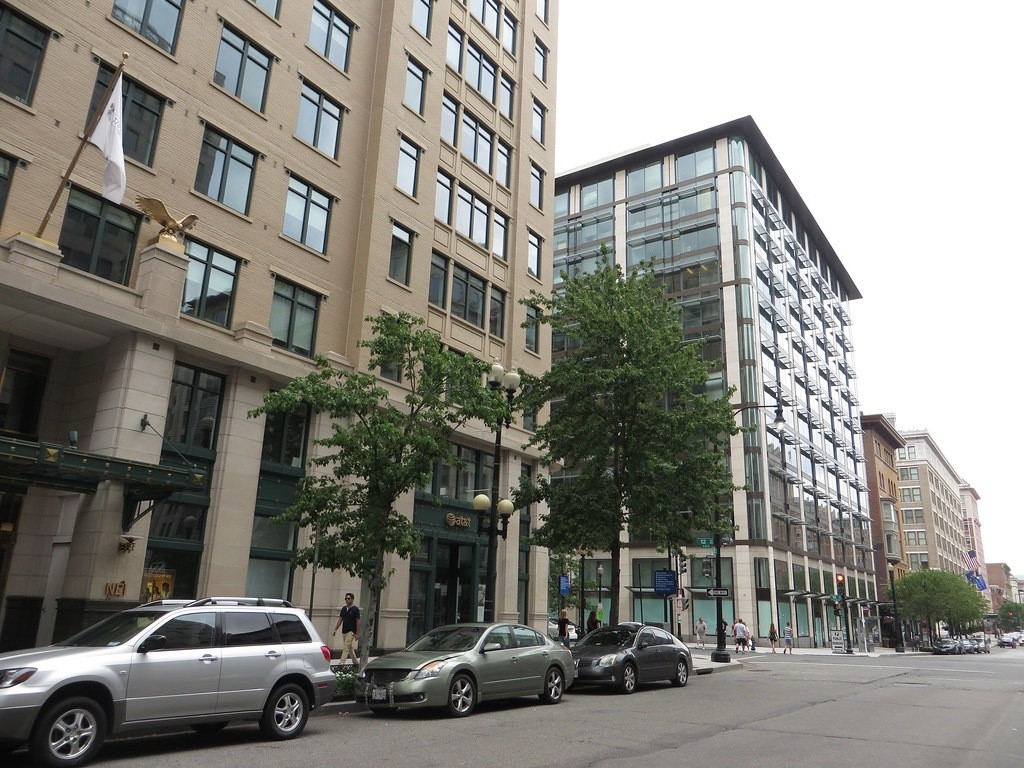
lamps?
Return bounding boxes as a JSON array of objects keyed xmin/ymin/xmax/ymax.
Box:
[
  {"xmin": 117, "ymin": 534, "xmax": 143, "ymax": 554},
  {"xmin": 67, "ymin": 430, "xmax": 79, "ymax": 450}
]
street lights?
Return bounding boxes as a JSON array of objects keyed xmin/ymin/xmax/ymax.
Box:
[
  {"xmin": 598, "ymin": 564, "xmax": 603, "ymax": 628},
  {"xmin": 474, "ymin": 357, "xmax": 521, "ymax": 622},
  {"xmin": 887, "ymin": 561, "xmax": 906, "ymax": 652},
  {"xmin": 711, "ymin": 403, "xmax": 785, "ymax": 663},
  {"xmin": 579, "ymin": 541, "xmax": 588, "ymax": 640}
]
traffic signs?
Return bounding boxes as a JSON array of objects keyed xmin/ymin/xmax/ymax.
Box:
[{"xmin": 705, "ymin": 588, "xmax": 728, "ymax": 598}]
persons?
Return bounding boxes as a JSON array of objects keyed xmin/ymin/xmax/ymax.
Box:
[
  {"xmin": 144, "ymin": 583, "xmax": 174, "ymax": 602},
  {"xmin": 783, "ymin": 623, "xmax": 792, "ymax": 654},
  {"xmin": 722, "ymin": 619, "xmax": 728, "ymax": 648},
  {"xmin": 914, "ymin": 634, "xmax": 920, "ymax": 650},
  {"xmin": 767, "ymin": 623, "xmax": 778, "ymax": 654},
  {"xmin": 730, "ymin": 618, "xmax": 751, "ymax": 654},
  {"xmin": 557, "ymin": 610, "xmax": 569, "ymax": 649},
  {"xmin": 587, "ymin": 611, "xmax": 601, "ymax": 633},
  {"xmin": 332, "ymin": 593, "xmax": 360, "ymax": 664},
  {"xmin": 696, "ymin": 617, "xmax": 707, "ymax": 649}
]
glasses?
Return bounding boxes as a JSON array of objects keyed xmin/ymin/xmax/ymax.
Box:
[{"xmin": 345, "ymin": 598, "xmax": 353, "ymax": 600}]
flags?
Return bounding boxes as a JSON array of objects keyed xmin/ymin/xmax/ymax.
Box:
[
  {"xmin": 975, "ymin": 575, "xmax": 987, "ymax": 591},
  {"xmin": 966, "ymin": 571, "xmax": 977, "ymax": 584},
  {"xmin": 963, "ymin": 551, "xmax": 980, "ymax": 569},
  {"xmin": 87, "ymin": 70, "xmax": 126, "ymax": 204}
]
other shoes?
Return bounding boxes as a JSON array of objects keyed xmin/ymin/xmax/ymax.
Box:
[
  {"xmin": 696, "ymin": 646, "xmax": 699, "ymax": 649},
  {"xmin": 736, "ymin": 648, "xmax": 738, "ymax": 653},
  {"xmin": 702, "ymin": 648, "xmax": 705, "ymax": 649}
]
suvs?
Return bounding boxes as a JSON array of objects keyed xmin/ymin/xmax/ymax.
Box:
[{"xmin": 0, "ymin": 596, "xmax": 337, "ymax": 768}]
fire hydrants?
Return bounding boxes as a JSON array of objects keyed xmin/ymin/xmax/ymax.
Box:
[{"xmin": 751, "ymin": 636, "xmax": 757, "ymax": 650}]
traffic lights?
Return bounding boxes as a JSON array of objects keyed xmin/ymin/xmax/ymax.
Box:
[
  {"xmin": 837, "ymin": 575, "xmax": 846, "ymax": 593},
  {"xmin": 1008, "ymin": 612, "xmax": 1013, "ymax": 617},
  {"xmin": 833, "ymin": 603, "xmax": 842, "ymax": 616}
]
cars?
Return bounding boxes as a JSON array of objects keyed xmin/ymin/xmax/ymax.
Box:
[
  {"xmin": 355, "ymin": 623, "xmax": 574, "ymax": 718},
  {"xmin": 547, "ymin": 616, "xmax": 586, "ymax": 641},
  {"xmin": 933, "ymin": 634, "xmax": 991, "ymax": 655},
  {"xmin": 569, "ymin": 622, "xmax": 694, "ymax": 694},
  {"xmin": 998, "ymin": 632, "xmax": 1024, "ymax": 648}
]
